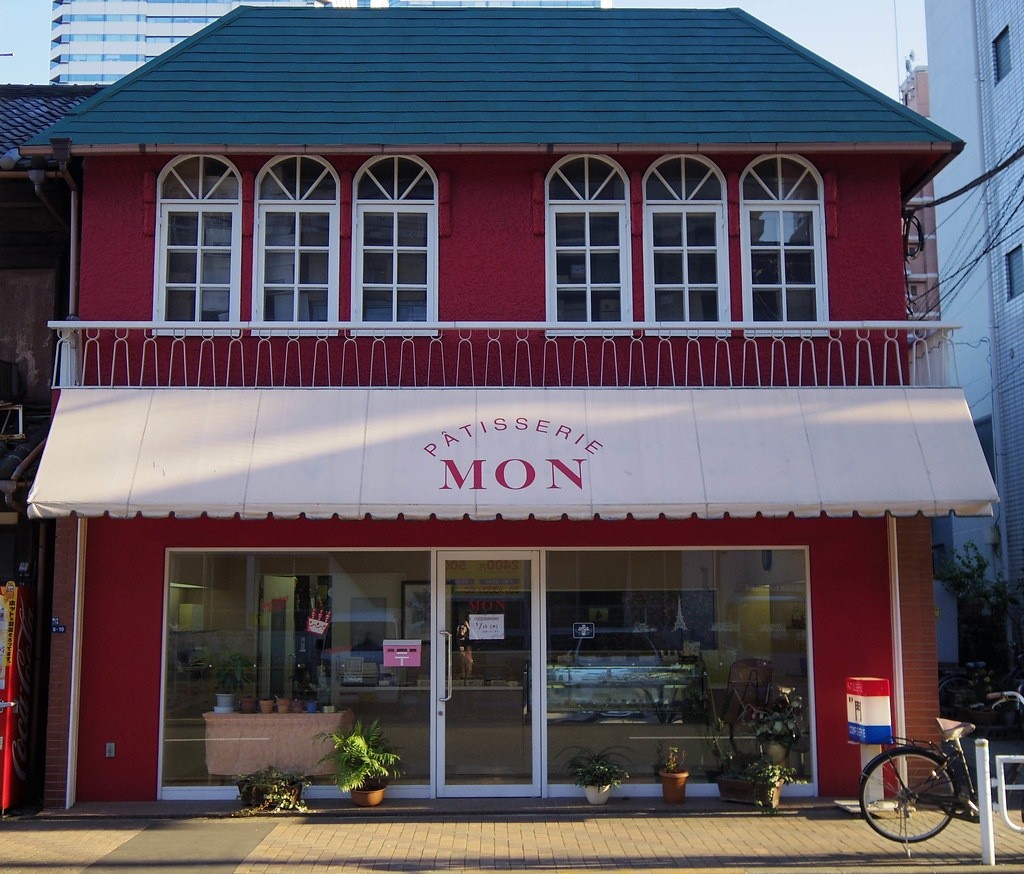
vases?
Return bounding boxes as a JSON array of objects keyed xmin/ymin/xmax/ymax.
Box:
[{"xmin": 240, "ymin": 696, "xmax": 338, "ymax": 713}]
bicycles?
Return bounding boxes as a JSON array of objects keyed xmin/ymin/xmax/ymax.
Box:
[
  {"xmin": 938, "ymin": 643, "xmax": 1024, "ymax": 737},
  {"xmin": 857, "ymin": 691, "xmax": 1024, "ymax": 854},
  {"xmin": 729, "ymin": 686, "xmax": 809, "ymax": 763}
]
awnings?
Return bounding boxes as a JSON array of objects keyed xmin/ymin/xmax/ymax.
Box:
[{"xmin": 28, "ymin": 386, "xmax": 1003, "ymax": 524}]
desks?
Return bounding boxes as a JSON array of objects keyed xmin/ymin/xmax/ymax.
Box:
[{"xmin": 202, "ymin": 708, "xmax": 354, "ymax": 785}]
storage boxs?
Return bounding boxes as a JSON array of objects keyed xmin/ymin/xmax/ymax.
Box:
[{"xmin": 178, "ymin": 603, "xmax": 204, "ymax": 632}]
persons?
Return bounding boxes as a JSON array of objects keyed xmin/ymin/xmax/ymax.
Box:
[{"xmin": 456, "ymin": 621, "xmax": 479, "ymax": 680}]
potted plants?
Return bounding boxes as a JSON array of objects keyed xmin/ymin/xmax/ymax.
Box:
[
  {"xmin": 739, "ymin": 693, "xmax": 804, "ymax": 762},
  {"xmin": 939, "ymin": 666, "xmax": 1019, "ymax": 725},
  {"xmin": 230, "ymin": 767, "xmax": 314, "ymax": 816},
  {"xmin": 717, "ymin": 755, "xmax": 808, "ymax": 818},
  {"xmin": 213, "ymin": 649, "xmax": 258, "ymax": 713},
  {"xmin": 658, "ymin": 746, "xmax": 690, "ymax": 802},
  {"xmin": 310, "ymin": 719, "xmax": 409, "ymax": 806},
  {"xmin": 552, "ymin": 746, "xmax": 636, "ymax": 805}
]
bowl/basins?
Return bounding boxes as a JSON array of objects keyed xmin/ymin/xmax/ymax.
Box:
[{"xmin": 324, "ymin": 706, "xmax": 334, "ymax": 712}]
